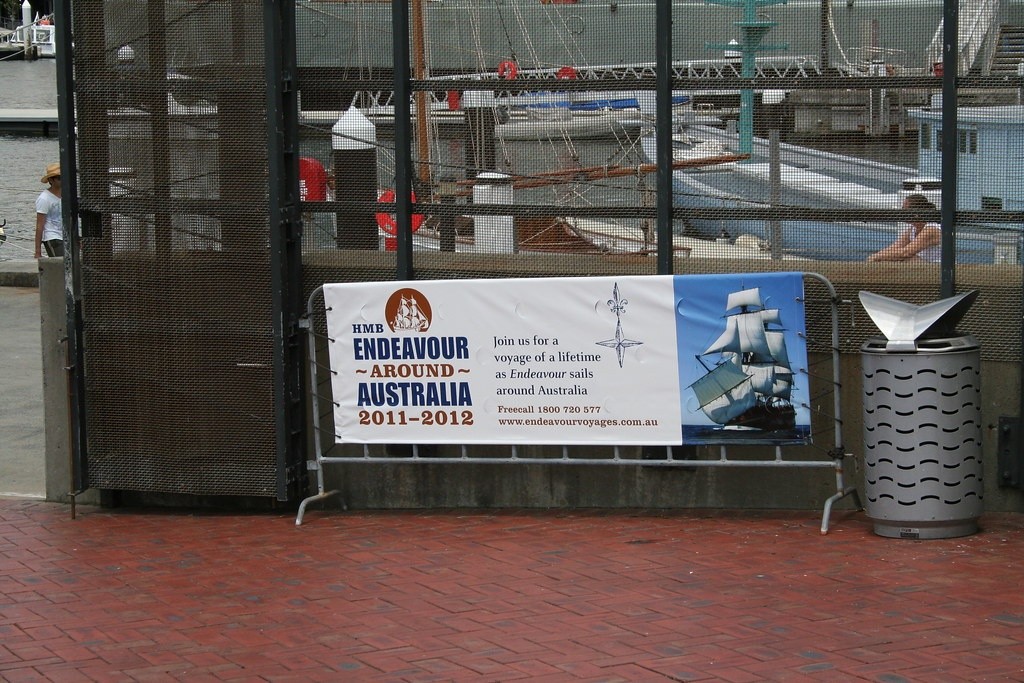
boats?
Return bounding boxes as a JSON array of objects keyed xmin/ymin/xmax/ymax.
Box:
[
  {"xmin": 9, "ymin": 23, "xmax": 56, "ymax": 58},
  {"xmin": 320, "ymin": 87, "xmax": 1024, "ymax": 267}
]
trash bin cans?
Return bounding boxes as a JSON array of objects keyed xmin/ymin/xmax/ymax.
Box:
[{"xmin": 858, "ymin": 289, "xmax": 983, "ymax": 540}]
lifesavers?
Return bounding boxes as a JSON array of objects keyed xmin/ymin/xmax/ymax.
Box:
[
  {"xmin": 558, "ymin": 67, "xmax": 576, "ymax": 80},
  {"xmin": 375, "ymin": 190, "xmax": 424, "ymax": 235},
  {"xmin": 498, "ymin": 61, "xmax": 516, "ymax": 80}
]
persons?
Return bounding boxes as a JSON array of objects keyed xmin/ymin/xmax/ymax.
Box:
[
  {"xmin": 34, "ymin": 164, "xmax": 64, "ymax": 259},
  {"xmin": 867, "ymin": 194, "xmax": 941, "ymax": 263}
]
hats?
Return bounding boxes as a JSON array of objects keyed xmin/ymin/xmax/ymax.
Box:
[{"xmin": 41, "ymin": 163, "xmax": 61, "ymax": 183}]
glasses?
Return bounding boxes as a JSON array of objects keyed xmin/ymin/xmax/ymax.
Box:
[{"xmin": 50, "ymin": 175, "xmax": 60, "ymax": 181}]
